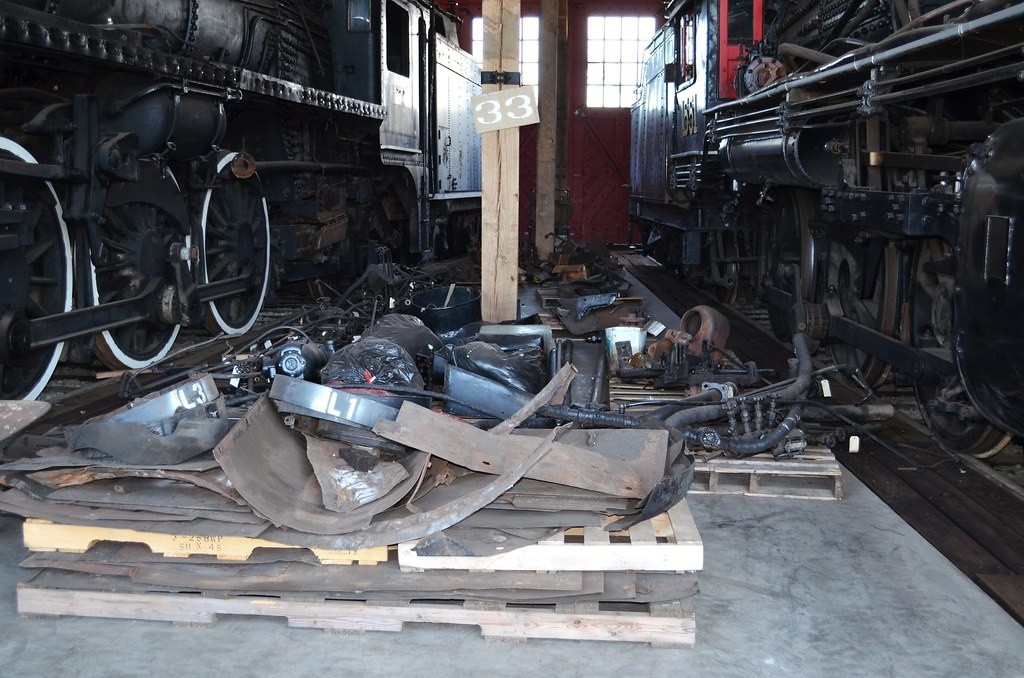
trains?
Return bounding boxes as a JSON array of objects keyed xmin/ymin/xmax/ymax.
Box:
[
  {"xmin": 627, "ymin": 0, "xmax": 1023, "ymax": 474},
  {"xmin": 1, "ymin": 0, "xmax": 482, "ymax": 402}
]
